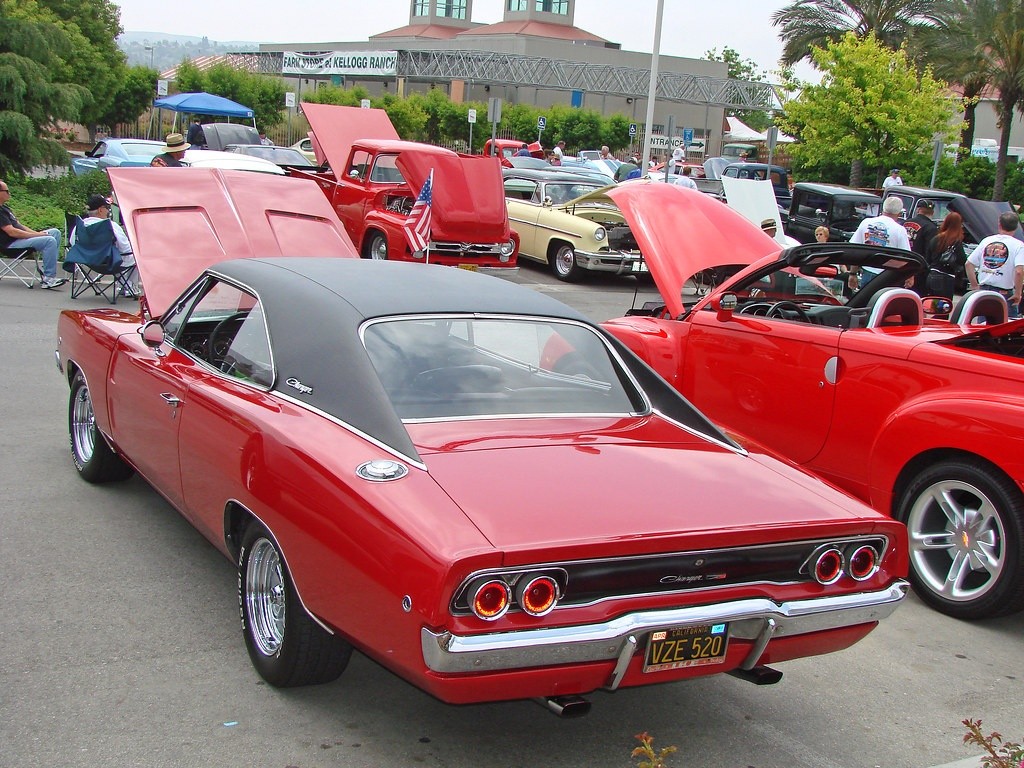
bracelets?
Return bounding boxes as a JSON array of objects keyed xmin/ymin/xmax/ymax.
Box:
[{"xmin": 850, "ymin": 272, "xmax": 857, "ymax": 276}]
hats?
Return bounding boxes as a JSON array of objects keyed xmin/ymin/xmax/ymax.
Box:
[
  {"xmin": 628, "ymin": 157, "xmax": 638, "ymax": 165},
  {"xmin": 739, "ymin": 152, "xmax": 748, "ymax": 156},
  {"xmin": 759, "ymin": 218, "xmax": 777, "ymax": 231},
  {"xmin": 161, "ymin": 132, "xmax": 191, "ymax": 153},
  {"xmin": 917, "ymin": 198, "xmax": 936, "ymax": 209},
  {"xmin": 652, "ymin": 156, "xmax": 659, "ymax": 159},
  {"xmin": 892, "ymin": 169, "xmax": 900, "ymax": 174},
  {"xmin": 86, "ymin": 193, "xmax": 110, "ymax": 211}
]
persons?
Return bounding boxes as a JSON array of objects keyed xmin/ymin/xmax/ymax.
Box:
[
  {"xmin": 648, "ymin": 156, "xmax": 659, "ymax": 168},
  {"xmin": 666, "ymin": 153, "xmax": 676, "ymax": 174},
  {"xmin": 673, "ymin": 143, "xmax": 686, "ymax": 162},
  {"xmin": 816, "ymin": 225, "xmax": 849, "ymax": 274},
  {"xmin": 902, "ymin": 198, "xmax": 1024, "ymax": 323},
  {"xmin": 634, "ymin": 151, "xmax": 642, "ymax": 169},
  {"xmin": 69, "ymin": 194, "xmax": 141, "ymax": 297},
  {"xmin": 759, "ymin": 218, "xmax": 806, "ymax": 298},
  {"xmin": 187, "ymin": 118, "xmax": 203, "ymax": 150},
  {"xmin": 737, "ymin": 152, "xmax": 749, "ymax": 161},
  {"xmin": 150, "ymin": 132, "xmax": 192, "ymax": 167},
  {"xmin": 845, "ymin": 197, "xmax": 916, "ymax": 292},
  {"xmin": 259, "ymin": 132, "xmax": 274, "ymax": 146},
  {"xmin": 552, "ymin": 141, "xmax": 566, "ymax": 156},
  {"xmin": 518, "ymin": 144, "xmax": 532, "ymax": 157},
  {"xmin": 600, "ymin": 145, "xmax": 616, "ymax": 159},
  {"xmin": 614, "ymin": 156, "xmax": 638, "ymax": 183},
  {"xmin": 674, "ymin": 167, "xmax": 698, "ymax": 191},
  {"xmin": 0, "ymin": 178, "xmax": 69, "ymax": 288},
  {"xmin": 881, "ymin": 168, "xmax": 904, "ymax": 190}
]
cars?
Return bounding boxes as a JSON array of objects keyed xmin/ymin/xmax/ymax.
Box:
[{"xmin": 65, "ymin": 100, "xmax": 1024, "ymax": 310}]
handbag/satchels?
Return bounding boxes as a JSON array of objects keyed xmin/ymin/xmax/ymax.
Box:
[{"xmin": 940, "ymin": 243, "xmax": 958, "ymax": 265}]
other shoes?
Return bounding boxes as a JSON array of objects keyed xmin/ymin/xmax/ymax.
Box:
[{"xmin": 116, "ymin": 286, "xmax": 143, "ymax": 297}]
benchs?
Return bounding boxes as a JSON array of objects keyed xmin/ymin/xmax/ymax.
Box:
[
  {"xmin": 388, "ymin": 386, "xmax": 592, "ymax": 406},
  {"xmin": 504, "ymin": 190, "xmax": 557, "ymax": 199}
]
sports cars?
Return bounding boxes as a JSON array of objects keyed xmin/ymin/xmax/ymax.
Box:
[
  {"xmin": 54, "ymin": 165, "xmax": 913, "ymax": 720},
  {"xmin": 584, "ymin": 180, "xmax": 1024, "ymax": 625}
]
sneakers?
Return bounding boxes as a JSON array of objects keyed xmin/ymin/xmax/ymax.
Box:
[{"xmin": 40, "ymin": 278, "xmax": 67, "ymax": 289}]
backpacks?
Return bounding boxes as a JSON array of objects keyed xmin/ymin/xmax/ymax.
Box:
[{"xmin": 193, "ymin": 128, "xmax": 206, "ymax": 145}]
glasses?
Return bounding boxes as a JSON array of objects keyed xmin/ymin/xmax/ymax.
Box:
[
  {"xmin": 815, "ymin": 233, "xmax": 822, "ymax": 236},
  {"xmin": 102, "ymin": 204, "xmax": 111, "ymax": 210},
  {"xmin": 0, "ymin": 190, "xmax": 10, "ymax": 194}
]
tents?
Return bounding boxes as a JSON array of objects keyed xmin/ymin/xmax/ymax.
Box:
[{"xmin": 146, "ymin": 92, "xmax": 256, "ymax": 141}]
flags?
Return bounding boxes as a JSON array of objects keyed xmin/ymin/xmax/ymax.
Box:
[{"xmin": 403, "ymin": 175, "xmax": 433, "ymax": 255}]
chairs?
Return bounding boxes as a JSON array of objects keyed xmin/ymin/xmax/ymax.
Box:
[
  {"xmin": 68, "ymin": 213, "xmax": 138, "ymax": 305},
  {"xmin": 0, "ymin": 247, "xmax": 43, "ymax": 288},
  {"xmin": 858, "ymin": 287, "xmax": 925, "ymax": 327},
  {"xmin": 950, "ymin": 286, "xmax": 1008, "ymax": 324},
  {"xmin": 409, "ymin": 364, "xmax": 501, "ymax": 387},
  {"xmin": 64, "ymin": 212, "xmax": 102, "ymax": 286}
]
